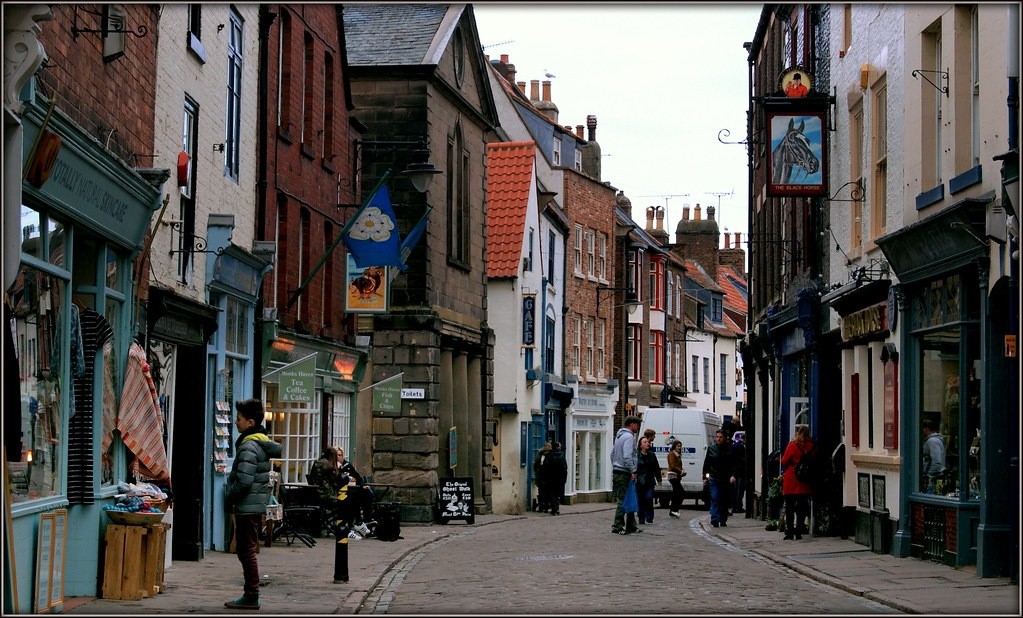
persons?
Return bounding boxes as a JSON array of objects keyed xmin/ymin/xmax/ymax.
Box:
[
  {"xmin": 303, "ymin": 446, "xmax": 375, "ymax": 541},
  {"xmin": 780, "ymin": 425, "xmax": 817, "ymax": 541},
  {"xmin": 666, "ymin": 440, "xmax": 686, "ymax": 517},
  {"xmin": 635, "ymin": 429, "xmax": 663, "ymax": 525},
  {"xmin": 609, "ymin": 415, "xmax": 645, "ymax": 534},
  {"xmin": 533, "ymin": 441, "xmax": 569, "ymax": 512},
  {"xmin": 702, "ymin": 429, "xmax": 749, "ymax": 527},
  {"xmin": 223, "ymin": 399, "xmax": 284, "ymax": 610},
  {"xmin": 922, "ymin": 419, "xmax": 947, "ymax": 495}
]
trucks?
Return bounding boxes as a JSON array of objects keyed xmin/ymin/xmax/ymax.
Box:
[{"xmin": 636, "ymin": 408, "xmax": 723, "ymax": 501}]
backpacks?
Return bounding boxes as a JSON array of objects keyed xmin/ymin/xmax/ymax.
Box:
[{"xmin": 790, "ymin": 441, "xmax": 822, "ymax": 486}]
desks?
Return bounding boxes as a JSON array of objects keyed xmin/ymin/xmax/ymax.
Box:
[
  {"xmin": 366, "ymin": 481, "xmax": 396, "ymax": 515},
  {"xmin": 281, "ymin": 482, "xmax": 320, "ymax": 544}
]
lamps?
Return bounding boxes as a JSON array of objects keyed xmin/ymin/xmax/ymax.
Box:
[
  {"xmin": 596, "ymin": 281, "xmax": 644, "ymax": 318},
  {"xmin": 820, "ymin": 280, "xmax": 863, "ymax": 302},
  {"xmin": 353, "ymin": 135, "xmax": 444, "ymax": 196}
]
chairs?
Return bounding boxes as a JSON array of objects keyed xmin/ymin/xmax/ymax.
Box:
[
  {"xmin": 275, "ymin": 473, "xmax": 402, "ymax": 549},
  {"xmin": 72, "ymin": 294, "xmax": 87, "ymax": 309}
]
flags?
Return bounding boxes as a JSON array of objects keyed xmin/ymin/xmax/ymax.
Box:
[
  {"xmin": 340, "ymin": 181, "xmax": 408, "ymax": 272},
  {"xmin": 370, "ymin": 374, "xmax": 403, "ymax": 413},
  {"xmin": 277, "ymin": 355, "xmax": 317, "ymax": 404}
]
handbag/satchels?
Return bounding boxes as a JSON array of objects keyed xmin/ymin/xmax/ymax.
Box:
[
  {"xmin": 622, "ymin": 477, "xmax": 638, "ymax": 512},
  {"xmin": 266, "ymin": 495, "xmax": 283, "ymax": 521},
  {"xmin": 374, "ymin": 510, "xmax": 404, "ymax": 542}
]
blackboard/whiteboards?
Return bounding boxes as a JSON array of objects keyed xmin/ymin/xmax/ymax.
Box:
[{"xmin": 438, "ymin": 476, "xmax": 475, "ymax": 525}]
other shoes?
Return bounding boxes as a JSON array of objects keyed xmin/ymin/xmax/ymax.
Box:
[
  {"xmin": 783, "ymin": 534, "xmax": 794, "ymax": 540},
  {"xmin": 224, "ymin": 590, "xmax": 260, "ymax": 608},
  {"xmin": 626, "ymin": 526, "xmax": 643, "ymax": 534},
  {"xmin": 669, "ymin": 510, "xmax": 680, "ymax": 518},
  {"xmin": 720, "ymin": 521, "xmax": 727, "ymax": 527},
  {"xmin": 796, "ymin": 535, "xmax": 802, "ymax": 539},
  {"xmin": 612, "ymin": 527, "xmax": 627, "ymax": 535},
  {"xmin": 710, "ymin": 519, "xmax": 720, "ymax": 527}
]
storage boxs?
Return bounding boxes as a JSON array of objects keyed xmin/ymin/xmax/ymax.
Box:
[{"xmin": 102, "ymin": 522, "xmax": 170, "ymax": 599}]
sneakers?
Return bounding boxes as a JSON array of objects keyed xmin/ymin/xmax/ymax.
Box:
[
  {"xmin": 348, "ymin": 530, "xmax": 362, "ymax": 540},
  {"xmin": 354, "ymin": 522, "xmax": 370, "ymax": 534}
]
